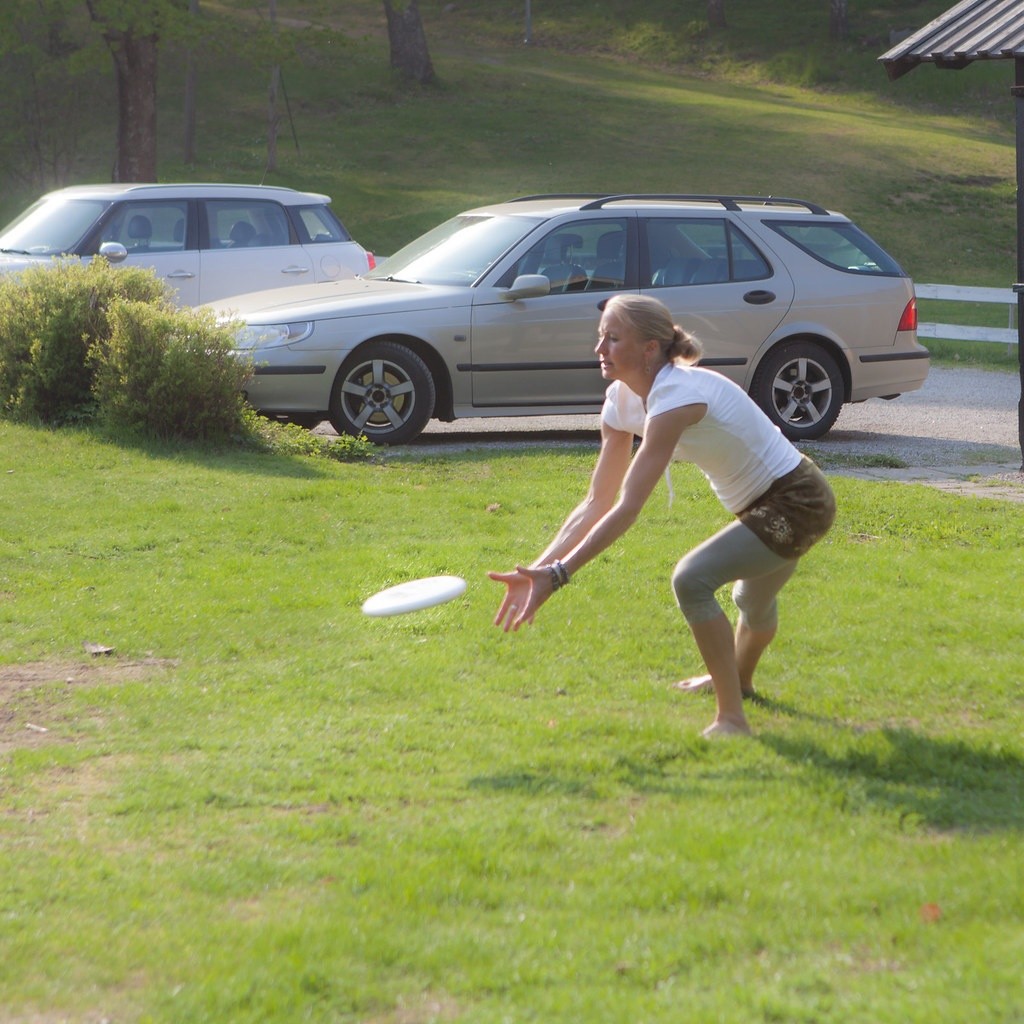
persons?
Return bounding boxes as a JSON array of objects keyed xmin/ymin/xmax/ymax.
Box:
[{"xmin": 487, "ymin": 293, "xmax": 836, "ymax": 741}]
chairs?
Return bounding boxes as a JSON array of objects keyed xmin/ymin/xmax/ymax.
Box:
[
  {"xmin": 125, "ymin": 215, "xmax": 153, "ymax": 254},
  {"xmin": 226, "ymin": 221, "xmax": 256, "ymax": 249},
  {"xmin": 584, "ymin": 231, "xmax": 627, "ymax": 290},
  {"xmin": 541, "ymin": 235, "xmax": 588, "ymax": 292}
]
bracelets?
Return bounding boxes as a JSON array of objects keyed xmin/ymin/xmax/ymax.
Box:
[{"xmin": 547, "ymin": 558, "xmax": 570, "ymax": 591}]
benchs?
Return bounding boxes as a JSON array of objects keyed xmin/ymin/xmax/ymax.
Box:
[{"xmin": 649, "ymin": 255, "xmax": 729, "ymax": 288}]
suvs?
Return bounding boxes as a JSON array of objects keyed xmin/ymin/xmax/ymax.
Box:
[
  {"xmin": 0, "ymin": 182, "xmax": 377, "ymax": 310},
  {"xmin": 189, "ymin": 193, "xmax": 932, "ymax": 444}
]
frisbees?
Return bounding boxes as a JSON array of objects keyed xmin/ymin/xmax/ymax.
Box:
[{"xmin": 360, "ymin": 572, "xmax": 467, "ymax": 619}]
